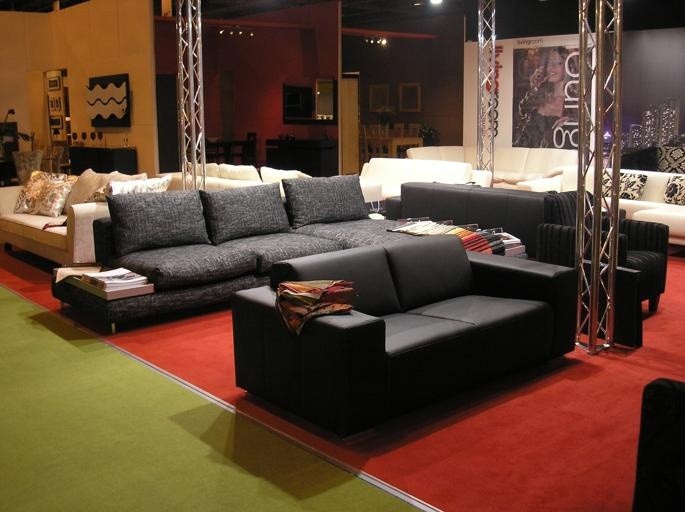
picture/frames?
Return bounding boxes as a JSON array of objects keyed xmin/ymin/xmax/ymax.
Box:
[
  {"xmin": 46, "ymin": 76, "xmax": 63, "ymax": 129},
  {"xmin": 368, "ymin": 84, "xmax": 391, "ymax": 113},
  {"xmin": 398, "ymin": 81, "xmax": 423, "ymax": 113}
]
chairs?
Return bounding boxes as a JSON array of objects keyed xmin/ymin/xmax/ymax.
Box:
[
  {"xmin": 359, "ymin": 122, "xmax": 424, "ymax": 173},
  {"xmin": 205, "ymin": 132, "xmax": 258, "ymax": 167}
]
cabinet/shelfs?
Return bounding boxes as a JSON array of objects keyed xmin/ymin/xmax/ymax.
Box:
[{"xmin": 265, "ymin": 138, "xmax": 338, "ymax": 178}]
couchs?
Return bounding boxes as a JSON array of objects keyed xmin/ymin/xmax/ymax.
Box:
[{"xmin": 629, "ymin": 374, "xmax": 684, "ymax": 511}]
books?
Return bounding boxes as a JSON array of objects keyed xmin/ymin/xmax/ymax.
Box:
[
  {"xmin": 51, "ymin": 263, "xmax": 155, "ymax": 301},
  {"xmin": 385, "ymin": 218, "xmax": 530, "ymax": 259}
]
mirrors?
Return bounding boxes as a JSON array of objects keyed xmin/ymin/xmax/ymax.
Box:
[{"xmin": 283, "ymin": 77, "xmax": 337, "ymax": 124}]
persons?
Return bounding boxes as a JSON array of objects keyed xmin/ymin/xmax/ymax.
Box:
[{"xmin": 515, "ymin": 48, "xmax": 588, "ymax": 146}]
